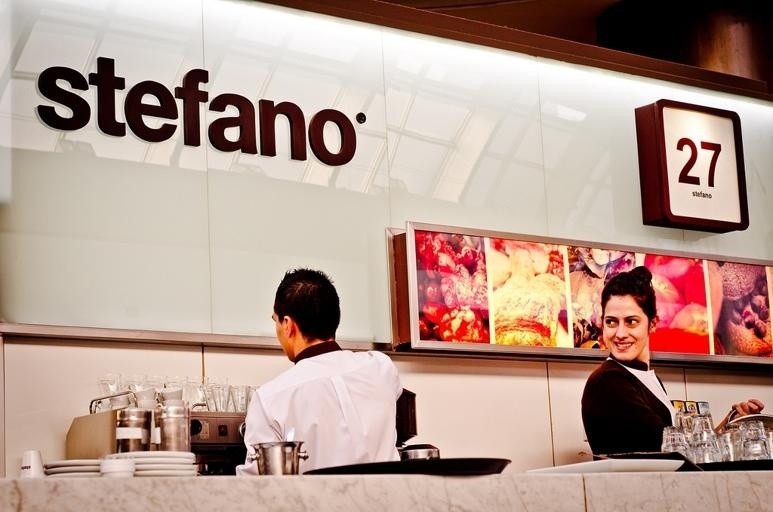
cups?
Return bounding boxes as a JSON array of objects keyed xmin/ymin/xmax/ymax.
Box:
[
  {"xmin": 92, "ymin": 372, "xmax": 262, "ymax": 412},
  {"xmin": 244, "ymin": 437, "xmax": 309, "ymax": 475},
  {"xmin": 659, "ymin": 412, "xmax": 772, "ymax": 464},
  {"xmin": 19, "ymin": 449, "xmax": 47, "ymax": 479}
]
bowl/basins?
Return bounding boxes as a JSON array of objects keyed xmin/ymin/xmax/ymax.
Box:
[{"xmin": 100, "ymin": 458, "xmax": 136, "ymax": 478}]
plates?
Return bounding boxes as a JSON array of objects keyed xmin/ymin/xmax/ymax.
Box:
[
  {"xmin": 43, "ymin": 459, "xmax": 102, "ymax": 478},
  {"xmin": 523, "ymin": 457, "xmax": 686, "ymax": 474},
  {"xmin": 103, "ymin": 450, "xmax": 200, "ymax": 476}
]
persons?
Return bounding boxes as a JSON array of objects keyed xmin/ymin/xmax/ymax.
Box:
[
  {"xmin": 234, "ymin": 265, "xmax": 404, "ymax": 475},
  {"xmin": 580, "ymin": 265, "xmax": 765, "ymax": 464}
]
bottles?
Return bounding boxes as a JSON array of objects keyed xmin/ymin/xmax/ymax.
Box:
[{"xmin": 116, "ymin": 407, "xmax": 193, "ymax": 452}]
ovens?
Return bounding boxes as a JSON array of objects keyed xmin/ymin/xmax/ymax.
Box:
[{"xmin": 62, "ymin": 408, "xmax": 246, "ymax": 473}]
